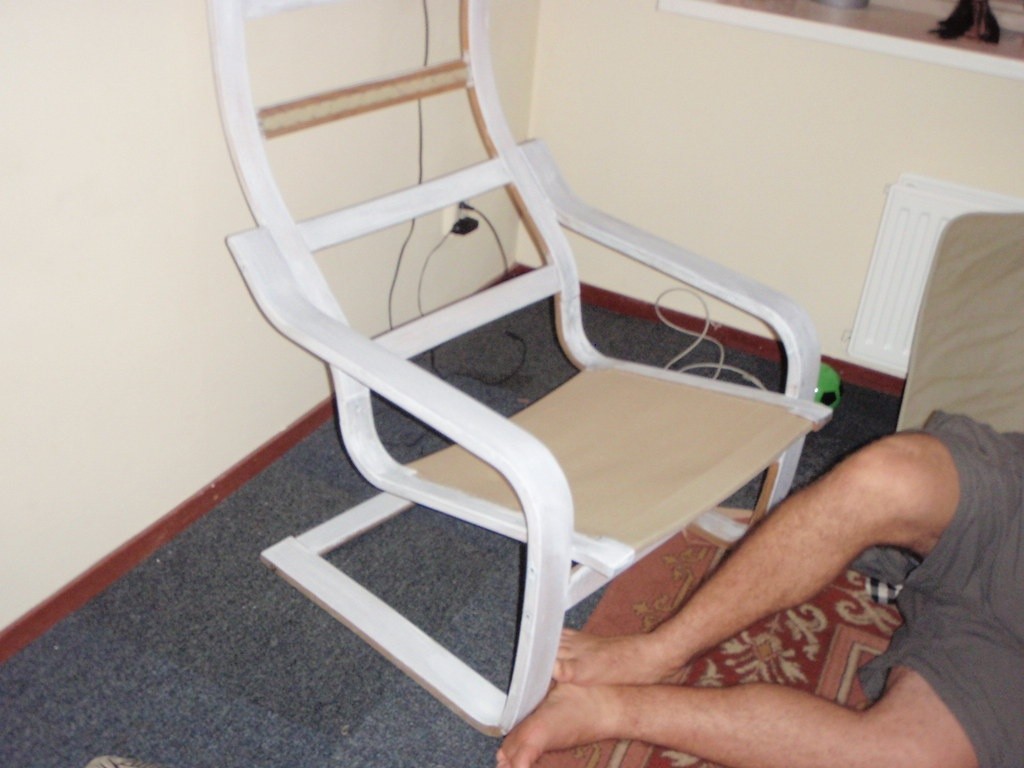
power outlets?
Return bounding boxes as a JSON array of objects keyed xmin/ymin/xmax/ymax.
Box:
[{"xmin": 446, "ymin": 191, "xmax": 472, "ymax": 236}]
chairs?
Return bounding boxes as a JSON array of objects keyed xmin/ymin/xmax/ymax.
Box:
[{"xmin": 204, "ymin": 0, "xmax": 836, "ymax": 738}]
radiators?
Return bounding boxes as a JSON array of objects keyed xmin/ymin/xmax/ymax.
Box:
[{"xmin": 845, "ymin": 173, "xmax": 1024, "ymax": 380}]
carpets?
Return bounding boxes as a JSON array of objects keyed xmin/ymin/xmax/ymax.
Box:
[{"xmin": 532, "ymin": 502, "xmax": 906, "ymax": 768}]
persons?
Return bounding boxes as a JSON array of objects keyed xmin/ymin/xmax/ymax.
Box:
[{"xmin": 497, "ymin": 411, "xmax": 1024, "ymax": 768}]
